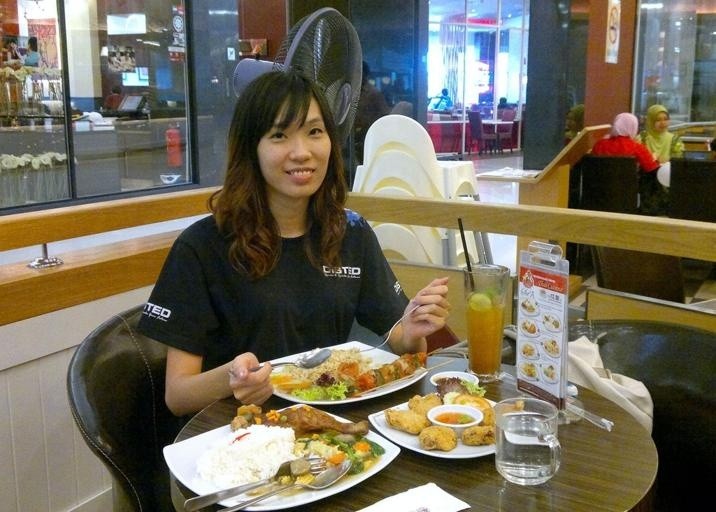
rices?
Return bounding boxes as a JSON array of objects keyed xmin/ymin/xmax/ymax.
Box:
[
  {"xmin": 282, "ymin": 346, "xmax": 375, "ymax": 398},
  {"xmin": 198, "ymin": 423, "xmax": 294, "ymax": 489}
]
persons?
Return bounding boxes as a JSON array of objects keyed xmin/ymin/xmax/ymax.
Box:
[
  {"xmin": 635, "ymin": 104, "xmax": 684, "ymax": 166},
  {"xmin": 103, "ymin": 83, "xmax": 125, "ymax": 111},
  {"xmin": 497, "ymin": 98, "xmax": 512, "ymax": 108},
  {"xmin": 591, "ymin": 112, "xmax": 664, "ymax": 211},
  {"xmin": 136, "ymin": 71, "xmax": 452, "ymax": 417},
  {"xmin": 434, "ymin": 87, "xmax": 453, "ymax": 111},
  {"xmin": 353, "ymin": 62, "xmax": 392, "ymax": 167},
  {"xmin": 20, "ymin": 34, "xmax": 39, "ymax": 66},
  {"xmin": 1, "ymin": 38, "xmax": 21, "ymax": 68}
]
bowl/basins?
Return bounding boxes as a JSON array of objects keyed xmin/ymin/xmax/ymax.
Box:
[
  {"xmin": 426, "ymin": 402, "xmax": 485, "ymax": 437},
  {"xmin": 430, "ymin": 370, "xmax": 480, "ymax": 388}
]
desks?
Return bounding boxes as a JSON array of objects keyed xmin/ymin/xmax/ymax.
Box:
[{"xmin": 483, "ymin": 119, "xmax": 513, "ymax": 156}]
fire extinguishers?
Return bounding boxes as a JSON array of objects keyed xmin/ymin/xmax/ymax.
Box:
[{"xmin": 166, "ymin": 123, "xmax": 181, "ymax": 168}]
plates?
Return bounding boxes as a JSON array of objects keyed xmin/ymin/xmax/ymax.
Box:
[
  {"xmin": 162, "ymin": 403, "xmax": 402, "ymax": 507},
  {"xmin": 367, "ymin": 391, "xmax": 498, "ymax": 460},
  {"xmin": 258, "ymin": 339, "xmax": 429, "ymax": 406}
]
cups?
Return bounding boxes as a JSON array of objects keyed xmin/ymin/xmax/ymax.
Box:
[
  {"xmin": 462, "ymin": 262, "xmax": 511, "ymax": 384},
  {"xmin": 493, "ymin": 397, "xmax": 563, "ymax": 487}
]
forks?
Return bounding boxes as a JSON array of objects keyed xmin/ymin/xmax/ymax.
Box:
[
  {"xmin": 356, "ymin": 304, "xmax": 423, "ymax": 355},
  {"xmin": 182, "ymin": 456, "xmax": 328, "ymax": 511}
]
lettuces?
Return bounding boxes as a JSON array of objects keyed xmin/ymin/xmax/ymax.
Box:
[
  {"xmin": 463, "ymin": 381, "xmax": 486, "ymax": 396},
  {"xmin": 296, "ymin": 382, "xmax": 350, "ymax": 402}
]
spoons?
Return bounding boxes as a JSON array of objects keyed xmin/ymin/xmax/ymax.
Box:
[
  {"xmin": 219, "ymin": 459, "xmax": 352, "ymax": 510},
  {"xmin": 247, "ymin": 348, "xmax": 331, "ymax": 374}
]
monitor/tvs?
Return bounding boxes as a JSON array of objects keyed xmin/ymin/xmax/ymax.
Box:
[{"xmin": 118, "ymin": 92, "xmax": 147, "ymax": 117}]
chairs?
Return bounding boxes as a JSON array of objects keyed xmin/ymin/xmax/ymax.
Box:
[
  {"xmin": 585, "ymin": 210, "xmax": 685, "ymax": 308},
  {"xmin": 65, "ymin": 301, "xmax": 170, "ymax": 512},
  {"xmin": 501, "ymin": 319, "xmax": 716, "ymax": 512},
  {"xmin": 668, "ymin": 158, "xmax": 716, "ymax": 224},
  {"xmin": 492, "ymin": 108, "xmax": 517, "ymax": 155},
  {"xmin": 344, "ymin": 114, "xmax": 493, "ymax": 272},
  {"xmin": 467, "ymin": 110, "xmax": 498, "ymax": 157},
  {"xmin": 581, "ymin": 153, "xmax": 662, "ymax": 216}
]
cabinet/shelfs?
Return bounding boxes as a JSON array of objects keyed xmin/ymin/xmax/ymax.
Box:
[{"xmin": 113, "ymin": 115, "xmax": 215, "ymax": 182}]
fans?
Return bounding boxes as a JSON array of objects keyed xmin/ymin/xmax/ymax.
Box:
[{"xmin": 232, "ymin": 6, "xmax": 363, "ymax": 151}]
